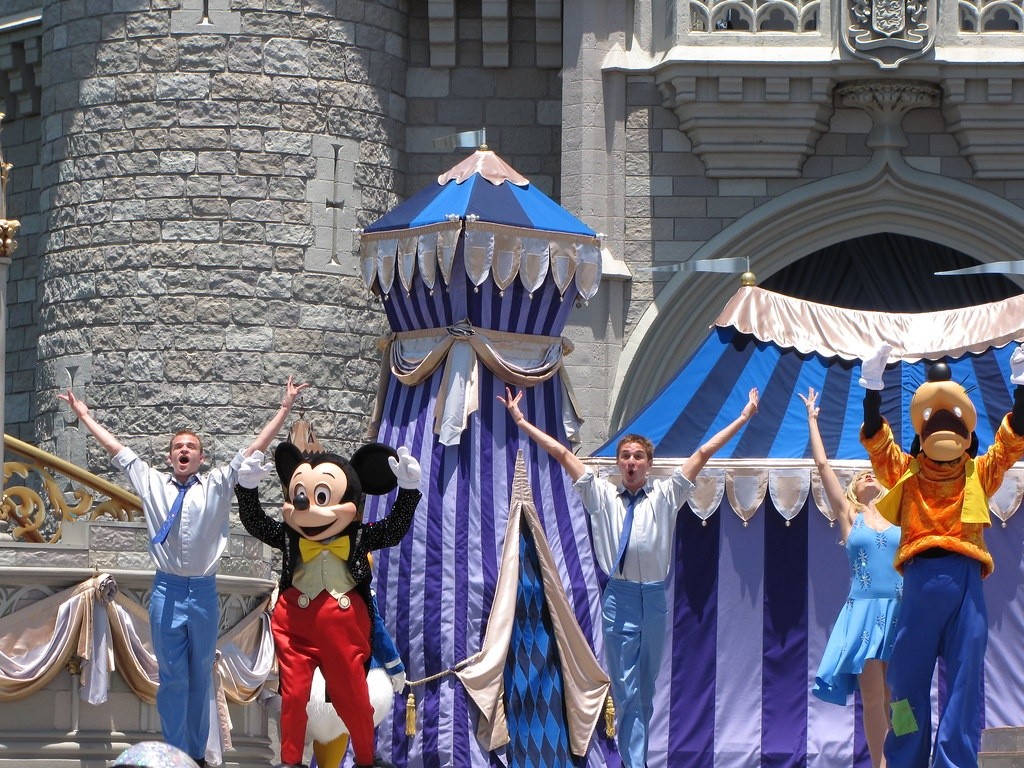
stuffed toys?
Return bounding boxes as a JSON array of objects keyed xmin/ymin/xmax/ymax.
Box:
[
  {"xmin": 859, "ymin": 340, "xmax": 1024, "ymax": 768},
  {"xmin": 235, "ymin": 442, "xmax": 423, "ymax": 768}
]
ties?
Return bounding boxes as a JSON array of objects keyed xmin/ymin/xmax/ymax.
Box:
[
  {"xmin": 150, "ymin": 479, "xmax": 198, "ymax": 545},
  {"xmin": 606, "ymin": 488, "xmax": 645, "ymax": 578}
]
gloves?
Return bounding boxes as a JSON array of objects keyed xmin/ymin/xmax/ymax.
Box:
[{"xmin": 391, "ymin": 671, "xmax": 407, "ymax": 695}]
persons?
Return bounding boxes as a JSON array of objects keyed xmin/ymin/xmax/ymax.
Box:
[
  {"xmin": 56, "ymin": 375, "xmax": 308, "ymax": 768},
  {"xmin": 498, "ymin": 386, "xmax": 759, "ymax": 768},
  {"xmin": 798, "ymin": 386, "xmax": 904, "ymax": 768}
]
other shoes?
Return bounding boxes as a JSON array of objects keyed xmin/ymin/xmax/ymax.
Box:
[{"xmin": 194, "ymin": 758, "xmax": 209, "ymax": 768}]
